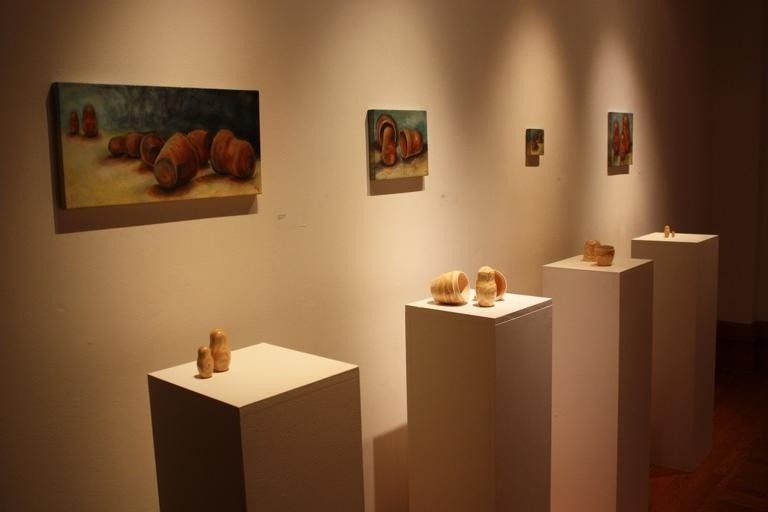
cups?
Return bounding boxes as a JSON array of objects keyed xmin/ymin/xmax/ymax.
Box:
[
  {"xmin": 376, "ymin": 114, "xmax": 423, "ymax": 160},
  {"xmin": 596, "ymin": 245, "xmax": 615, "ymax": 266},
  {"xmin": 141, "ymin": 129, "xmax": 256, "ymax": 192},
  {"xmin": 430, "ymin": 271, "xmax": 471, "ymax": 304}
]
